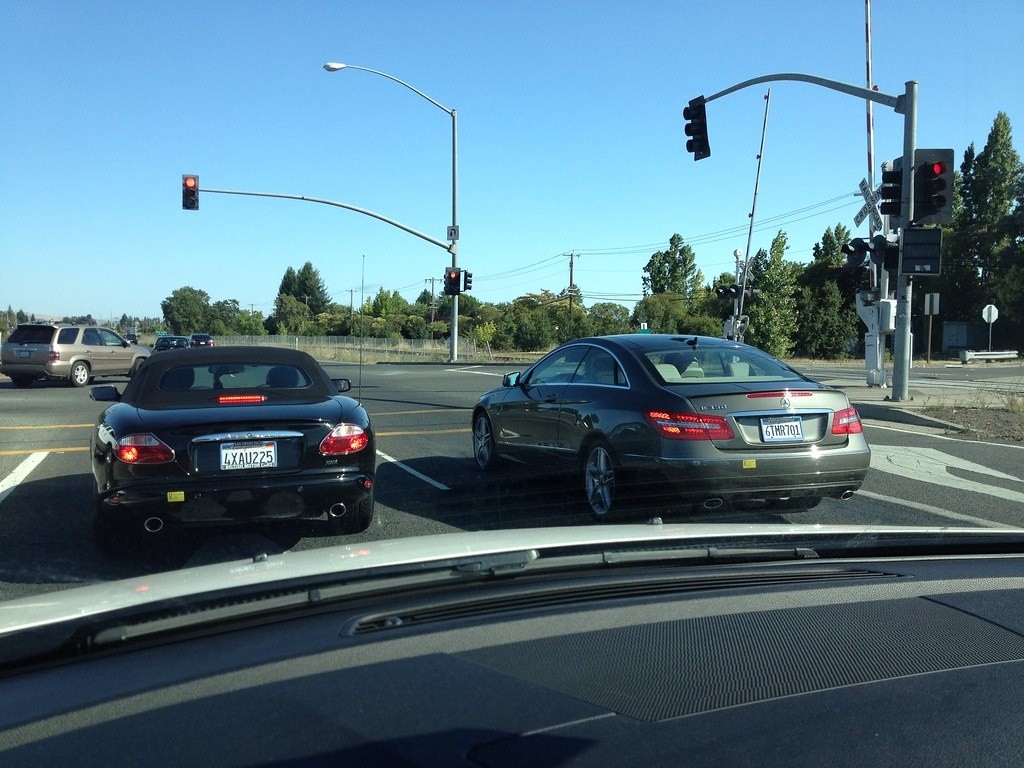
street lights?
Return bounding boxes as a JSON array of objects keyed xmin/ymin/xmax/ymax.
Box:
[{"xmin": 324, "ymin": 61, "xmax": 460, "ymax": 363}]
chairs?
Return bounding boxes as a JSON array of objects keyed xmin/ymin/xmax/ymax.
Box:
[
  {"xmin": 161, "ymin": 340, "xmax": 170, "ymax": 345},
  {"xmin": 725, "ymin": 363, "xmax": 756, "ymax": 377},
  {"xmin": 177, "ymin": 340, "xmax": 184, "ymax": 345},
  {"xmin": 654, "ymin": 363, "xmax": 681, "ymax": 380},
  {"xmin": 266, "ymin": 366, "xmax": 299, "ymax": 388},
  {"xmin": 681, "ymin": 368, "xmax": 704, "ymax": 379},
  {"xmin": 163, "ymin": 367, "xmax": 195, "ymax": 390}
]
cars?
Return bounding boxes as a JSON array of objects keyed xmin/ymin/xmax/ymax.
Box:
[
  {"xmin": 88, "ymin": 344, "xmax": 379, "ymax": 538},
  {"xmin": 149, "ymin": 335, "xmax": 191, "ymax": 349},
  {"xmin": 189, "ymin": 334, "xmax": 215, "ymax": 348},
  {"xmin": 126, "ymin": 334, "xmax": 138, "ymax": 346},
  {"xmin": 470, "ymin": 333, "xmax": 873, "ymax": 522},
  {"xmin": 151, "ymin": 345, "xmax": 188, "ymax": 351}
]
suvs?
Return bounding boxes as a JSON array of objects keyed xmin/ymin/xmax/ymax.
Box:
[{"xmin": 0, "ymin": 320, "xmax": 152, "ymax": 388}]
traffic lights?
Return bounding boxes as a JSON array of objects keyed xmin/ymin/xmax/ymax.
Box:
[
  {"xmin": 863, "ymin": 235, "xmax": 887, "ymax": 264},
  {"xmin": 881, "ymin": 166, "xmax": 902, "ymax": 217},
  {"xmin": 914, "ymin": 158, "xmax": 948, "ymax": 216},
  {"xmin": 446, "ymin": 267, "xmax": 461, "ymax": 296},
  {"xmin": 715, "ymin": 284, "xmax": 729, "ymax": 300},
  {"xmin": 443, "ymin": 274, "xmax": 447, "ymax": 292},
  {"xmin": 463, "ymin": 271, "xmax": 473, "ymax": 292},
  {"xmin": 682, "ymin": 95, "xmax": 711, "ymax": 161},
  {"xmin": 727, "ymin": 284, "xmax": 741, "ymax": 300},
  {"xmin": 840, "ymin": 238, "xmax": 868, "ymax": 267},
  {"xmin": 182, "ymin": 173, "xmax": 200, "ymax": 210}
]
